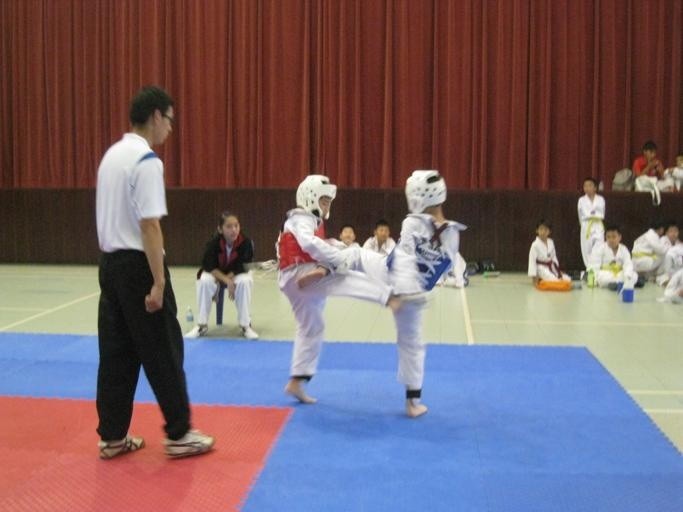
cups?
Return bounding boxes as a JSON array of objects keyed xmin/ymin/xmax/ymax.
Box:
[{"xmin": 622, "ymin": 288, "xmax": 634, "ymax": 302}]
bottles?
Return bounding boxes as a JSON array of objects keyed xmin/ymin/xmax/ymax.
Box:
[
  {"xmin": 587, "ymin": 268, "xmax": 595, "ymax": 288},
  {"xmin": 598, "ymin": 181, "xmax": 604, "ymax": 192},
  {"xmin": 186, "ymin": 307, "xmax": 193, "ymax": 332},
  {"xmin": 483, "ymin": 272, "xmax": 499, "ymax": 278}
]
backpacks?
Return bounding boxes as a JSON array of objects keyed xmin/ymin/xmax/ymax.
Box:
[{"xmin": 611, "ymin": 167, "xmax": 634, "ymax": 192}]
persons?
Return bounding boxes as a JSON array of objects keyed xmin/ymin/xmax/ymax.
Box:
[
  {"xmin": 328, "ymin": 224, "xmax": 361, "ymax": 250},
  {"xmin": 183, "ymin": 210, "xmax": 259, "ymax": 340},
  {"xmin": 362, "ymin": 220, "xmax": 397, "ymax": 256},
  {"xmin": 577, "ymin": 177, "xmax": 605, "ymax": 268},
  {"xmin": 297, "ymin": 169, "xmax": 469, "ymax": 416},
  {"xmin": 586, "ymin": 140, "xmax": 683, "ymax": 303},
  {"xmin": 275, "ymin": 174, "xmax": 404, "ymax": 405},
  {"xmin": 528, "ymin": 219, "xmax": 572, "ymax": 286},
  {"xmin": 95, "ymin": 85, "xmax": 214, "ymax": 458}
]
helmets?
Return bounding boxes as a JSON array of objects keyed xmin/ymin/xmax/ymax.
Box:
[
  {"xmin": 295, "ymin": 174, "xmax": 338, "ymax": 222},
  {"xmin": 404, "ymin": 168, "xmax": 447, "ymax": 214}
]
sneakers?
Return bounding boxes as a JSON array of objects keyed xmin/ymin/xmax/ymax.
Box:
[
  {"xmin": 98, "ymin": 434, "xmax": 146, "ymax": 460},
  {"xmin": 182, "ymin": 324, "xmax": 209, "ymax": 339},
  {"xmin": 239, "ymin": 325, "xmax": 259, "ymax": 341},
  {"xmin": 161, "ymin": 429, "xmax": 216, "ymax": 460}
]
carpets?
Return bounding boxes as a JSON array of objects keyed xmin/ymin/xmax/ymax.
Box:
[{"xmin": 0, "ymin": 332, "xmax": 683, "ymax": 512}]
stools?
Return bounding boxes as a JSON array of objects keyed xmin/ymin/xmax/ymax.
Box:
[{"xmin": 215, "ymin": 284, "xmax": 227, "ymax": 324}]
